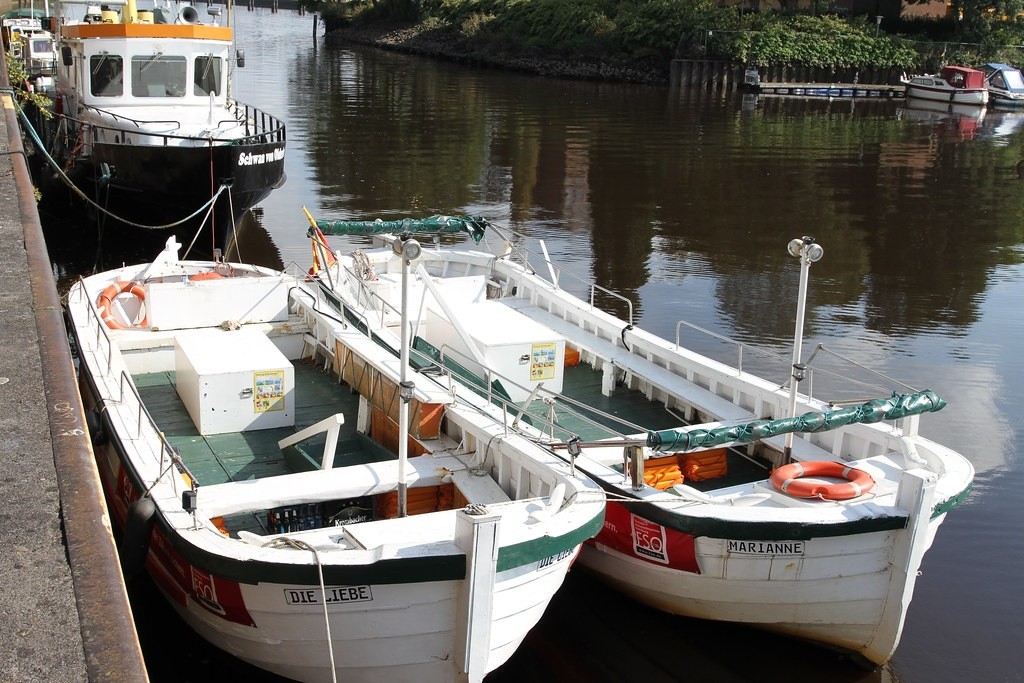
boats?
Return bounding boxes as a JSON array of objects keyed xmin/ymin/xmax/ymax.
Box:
[
  {"xmin": 976, "ymin": 63, "xmax": 1024, "ymax": 108},
  {"xmin": 311, "ymin": 210, "xmax": 974, "ymax": 666},
  {"xmin": 899, "ymin": 65, "xmax": 989, "ymax": 106},
  {"xmin": 0, "ymin": 0, "xmax": 287, "ymax": 222},
  {"xmin": 66, "ymin": 232, "xmax": 607, "ymax": 683}
]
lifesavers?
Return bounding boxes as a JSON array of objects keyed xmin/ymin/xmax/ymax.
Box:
[
  {"xmin": 97, "ymin": 280, "xmax": 148, "ymax": 331},
  {"xmin": 770, "ymin": 460, "xmax": 876, "ymax": 502}
]
